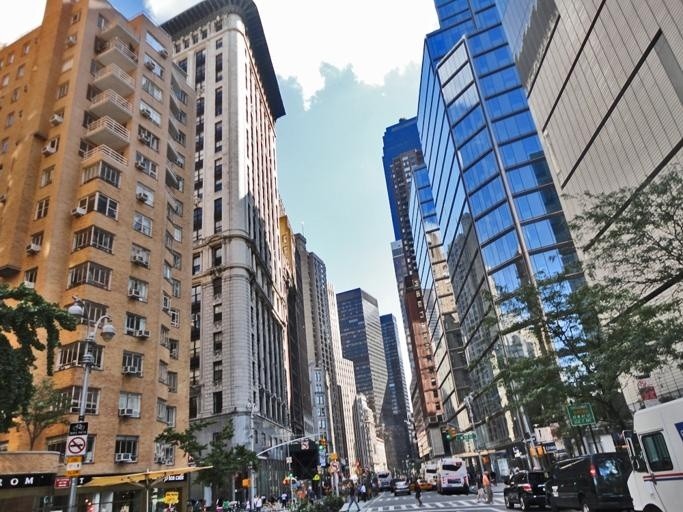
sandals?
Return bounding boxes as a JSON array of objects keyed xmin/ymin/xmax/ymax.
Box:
[
  {"xmin": 420, "ymin": 458, "xmax": 469, "ymax": 494},
  {"xmin": 377, "ymin": 471, "xmax": 392, "ymax": 491}
]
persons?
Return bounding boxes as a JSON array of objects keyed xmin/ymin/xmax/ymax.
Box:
[
  {"xmin": 482, "ymin": 470, "xmax": 493, "ymax": 503},
  {"xmin": 191, "ymin": 497, "xmax": 201, "ymax": 511},
  {"xmin": 253, "ymin": 492, "xmax": 288, "ymax": 512},
  {"xmin": 413, "ymin": 480, "xmax": 424, "ymax": 507},
  {"xmin": 214, "ymin": 498, "xmax": 224, "ymax": 512},
  {"xmin": 344, "ymin": 481, "xmax": 361, "ymax": 512},
  {"xmin": 473, "ymin": 471, "xmax": 486, "ymax": 503}
]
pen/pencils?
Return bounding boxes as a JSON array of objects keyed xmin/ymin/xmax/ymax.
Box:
[{"xmin": 545, "ymin": 452, "xmax": 633, "ymax": 512}]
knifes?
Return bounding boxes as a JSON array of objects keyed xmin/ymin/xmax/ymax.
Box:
[
  {"xmin": 68, "ymin": 207, "xmax": 86, "ymax": 216},
  {"xmin": 23, "ymin": 243, "xmax": 39, "ymax": 255},
  {"xmin": 134, "ymin": 47, "xmax": 169, "ymax": 202},
  {"xmin": 114, "ymin": 255, "xmax": 151, "ymax": 465},
  {"xmin": 39, "ymin": 112, "xmax": 60, "ymax": 156}
]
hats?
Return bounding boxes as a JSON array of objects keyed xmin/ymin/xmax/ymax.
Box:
[
  {"xmin": 503, "ymin": 470, "xmax": 549, "ymax": 511},
  {"xmin": 392, "ymin": 478, "xmax": 432, "ymax": 496}
]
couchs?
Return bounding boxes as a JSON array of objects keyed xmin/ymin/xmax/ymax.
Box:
[
  {"xmin": 456, "ymin": 433, "xmax": 477, "ymax": 440},
  {"xmin": 239, "ymin": 472, "xmax": 249, "ymax": 489},
  {"xmin": 319, "ymin": 432, "xmax": 328, "ymax": 447},
  {"xmin": 446, "ymin": 426, "xmax": 457, "ymax": 439}
]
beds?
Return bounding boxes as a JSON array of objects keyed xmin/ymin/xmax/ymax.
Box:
[{"xmin": 81, "ymin": 465, "xmax": 214, "ymax": 512}]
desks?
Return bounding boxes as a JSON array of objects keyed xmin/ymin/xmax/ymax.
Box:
[{"xmin": 68, "ymin": 295, "xmax": 116, "ymax": 512}]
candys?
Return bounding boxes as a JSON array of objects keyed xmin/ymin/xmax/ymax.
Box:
[{"xmin": 626, "ymin": 386, "xmax": 683, "ymax": 512}]
